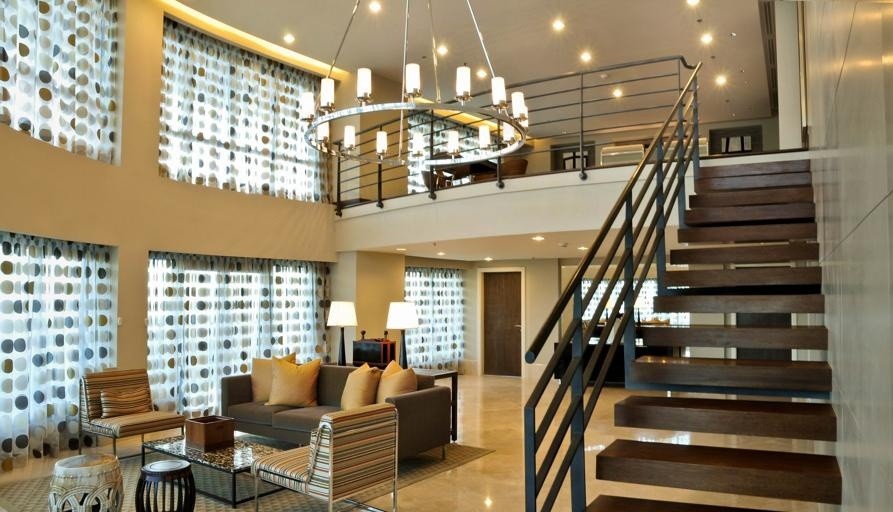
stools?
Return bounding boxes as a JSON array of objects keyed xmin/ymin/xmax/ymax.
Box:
[
  {"xmin": 49, "ymin": 452, "xmax": 125, "ymax": 512},
  {"xmin": 135, "ymin": 458, "xmax": 196, "ymax": 512}
]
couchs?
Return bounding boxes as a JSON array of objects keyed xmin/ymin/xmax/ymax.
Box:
[{"xmin": 220, "ymin": 364, "xmax": 413, "ymax": 463}]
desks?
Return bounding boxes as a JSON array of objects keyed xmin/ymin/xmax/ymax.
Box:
[
  {"xmin": 412, "ymin": 368, "xmax": 459, "ymax": 442},
  {"xmin": 554, "ymin": 337, "xmax": 658, "ymax": 388}
]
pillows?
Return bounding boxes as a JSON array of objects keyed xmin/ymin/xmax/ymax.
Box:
[
  {"xmin": 376, "ymin": 360, "xmax": 414, "ymax": 404},
  {"xmin": 264, "ymin": 355, "xmax": 322, "ymax": 407},
  {"xmin": 340, "ymin": 362, "xmax": 381, "ymax": 412},
  {"xmin": 99, "ymin": 380, "xmax": 151, "ymax": 420},
  {"xmin": 309, "ymin": 428, "xmax": 317, "ymax": 471},
  {"xmin": 251, "ymin": 351, "xmax": 296, "ymax": 404}
]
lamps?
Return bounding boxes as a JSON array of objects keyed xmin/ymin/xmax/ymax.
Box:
[
  {"xmin": 386, "ymin": 302, "xmax": 419, "ymax": 369},
  {"xmin": 326, "ymin": 300, "xmax": 358, "ymax": 366},
  {"xmin": 299, "ymin": 1, "xmax": 530, "ymax": 167}
]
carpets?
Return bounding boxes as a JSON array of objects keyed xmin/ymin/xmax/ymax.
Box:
[{"xmin": 0, "ymin": 441, "xmax": 496, "ymax": 512}]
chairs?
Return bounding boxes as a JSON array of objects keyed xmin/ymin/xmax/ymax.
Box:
[
  {"xmin": 421, "ymin": 170, "xmax": 454, "ymax": 192},
  {"xmin": 498, "ymin": 159, "xmax": 528, "ymax": 175},
  {"xmin": 78, "ymin": 368, "xmax": 186, "ymax": 457},
  {"xmin": 470, "ymin": 172, "xmax": 498, "ymax": 185},
  {"xmin": 250, "ymin": 402, "xmax": 399, "ymax": 511}
]
